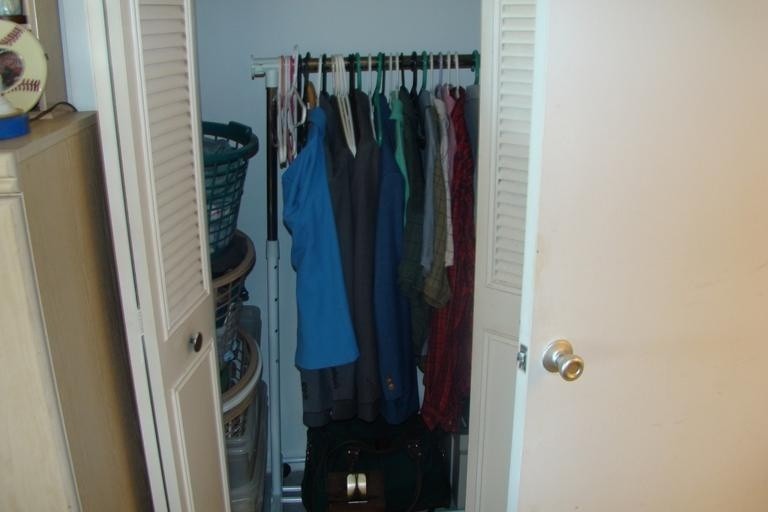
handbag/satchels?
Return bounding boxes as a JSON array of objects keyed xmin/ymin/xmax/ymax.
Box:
[{"xmin": 324, "ymin": 431, "xmax": 384, "ymax": 512}]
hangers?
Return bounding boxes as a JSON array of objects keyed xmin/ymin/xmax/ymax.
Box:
[
  {"xmin": 278, "ymin": 49, "xmax": 481, "ymax": 168},
  {"xmin": 249, "ymin": 52, "xmax": 476, "ymax": 511}
]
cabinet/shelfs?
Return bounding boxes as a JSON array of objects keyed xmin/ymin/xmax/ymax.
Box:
[{"xmin": 0, "ymin": 107, "xmax": 152, "ymax": 512}]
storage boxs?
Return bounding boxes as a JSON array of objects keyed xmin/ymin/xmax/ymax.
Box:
[{"xmin": 226, "ymin": 379, "xmax": 269, "ymax": 512}]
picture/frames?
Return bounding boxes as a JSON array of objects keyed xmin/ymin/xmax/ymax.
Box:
[{"xmin": 1, "ymin": 19, "xmax": 48, "ymax": 119}]
